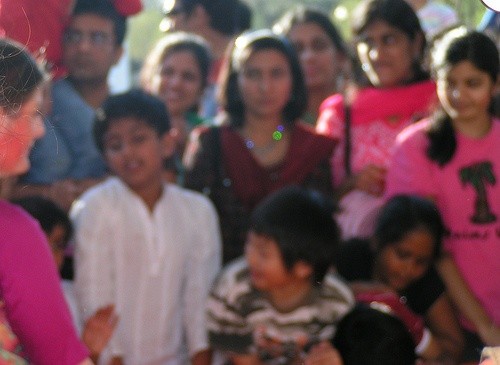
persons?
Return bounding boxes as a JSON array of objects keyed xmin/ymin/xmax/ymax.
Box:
[{"xmin": 0, "ymin": 0, "xmax": 500, "ymax": 365}]
